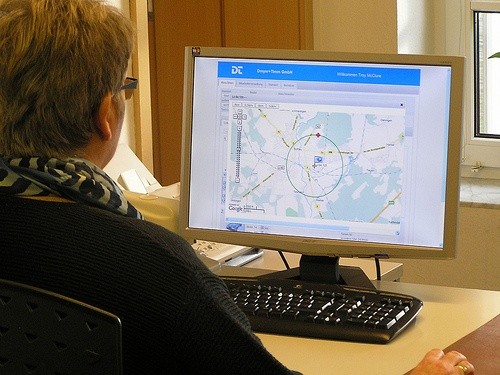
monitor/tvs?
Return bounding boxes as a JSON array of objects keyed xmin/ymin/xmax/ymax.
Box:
[{"xmin": 182, "ymin": 47, "xmax": 467, "ymax": 290}]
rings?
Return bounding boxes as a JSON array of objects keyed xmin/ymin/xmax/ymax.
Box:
[{"xmin": 455, "ymin": 364, "xmax": 468, "ymax": 374}]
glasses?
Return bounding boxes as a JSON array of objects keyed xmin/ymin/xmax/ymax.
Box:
[{"xmin": 111, "ymin": 76, "xmax": 138, "ymax": 100}]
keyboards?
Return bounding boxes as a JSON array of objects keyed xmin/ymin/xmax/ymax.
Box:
[{"xmin": 225, "ymin": 283, "xmax": 423, "ymax": 344}]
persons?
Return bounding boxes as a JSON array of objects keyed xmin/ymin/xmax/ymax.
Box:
[{"xmin": 0, "ymin": 0, "xmax": 476, "ymax": 375}]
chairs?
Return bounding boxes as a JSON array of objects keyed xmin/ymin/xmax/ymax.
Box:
[{"xmin": 0, "ymin": 278, "xmax": 125, "ymax": 375}]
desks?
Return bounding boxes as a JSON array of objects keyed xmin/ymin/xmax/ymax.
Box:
[{"xmin": 207, "ymin": 249, "xmax": 500, "ymax": 375}]
